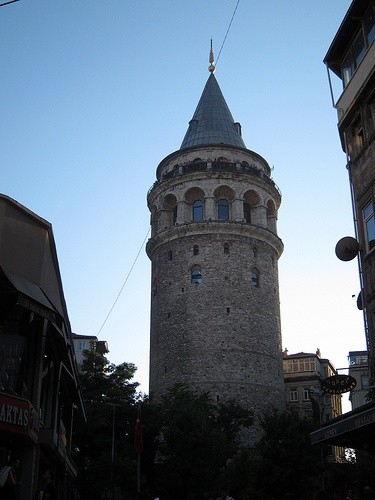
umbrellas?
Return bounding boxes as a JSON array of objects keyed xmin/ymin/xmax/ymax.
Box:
[{"xmin": 132, "ymin": 407, "xmax": 144, "ymax": 456}]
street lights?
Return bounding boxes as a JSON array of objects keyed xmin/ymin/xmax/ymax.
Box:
[{"xmin": 65, "ymin": 402, "xmax": 78, "ymax": 500}]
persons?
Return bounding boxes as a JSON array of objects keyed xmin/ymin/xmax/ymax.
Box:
[
  {"xmin": 359, "ymin": 486, "xmax": 375, "ymax": 500},
  {"xmin": 132, "ymin": 492, "xmax": 141, "ymax": 500},
  {"xmin": 342, "ymin": 488, "xmax": 360, "ymax": 500},
  {"xmin": 0, "ymin": 454, "xmax": 21, "ymax": 500},
  {"xmin": 41, "ymin": 467, "xmax": 57, "ymax": 500},
  {"xmin": 139, "ymin": 488, "xmax": 151, "ymax": 500},
  {"xmin": 312, "ymin": 489, "xmax": 331, "ymax": 500},
  {"xmin": 215, "ymin": 485, "xmax": 235, "ymax": 500}
]
